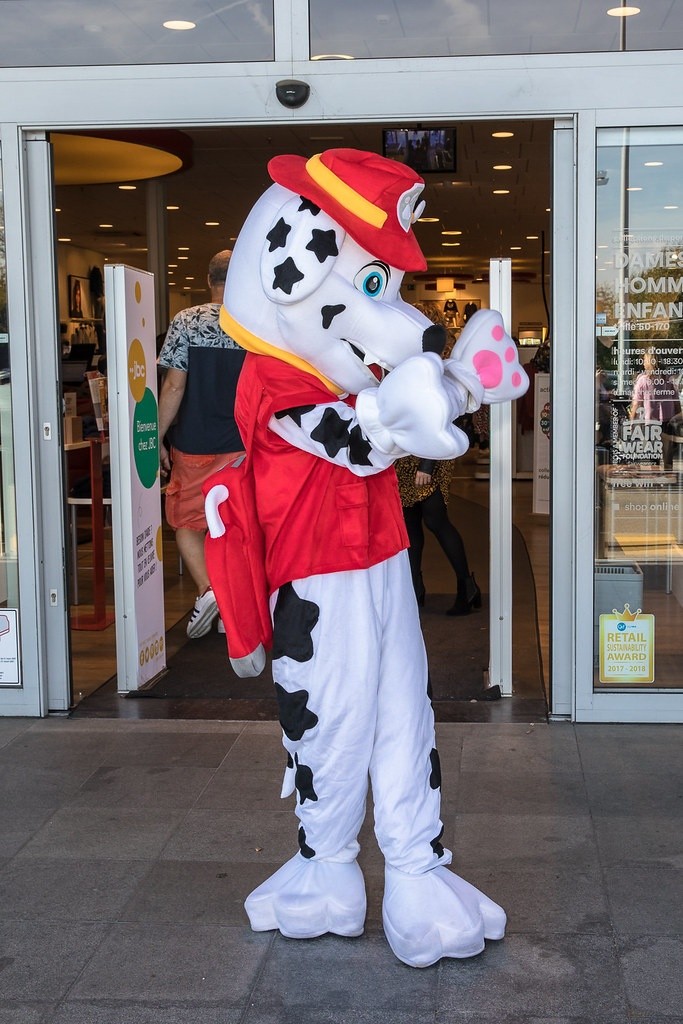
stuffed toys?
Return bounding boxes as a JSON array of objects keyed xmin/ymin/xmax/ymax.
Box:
[{"xmin": 202, "ymin": 147, "xmax": 538, "ymax": 970}]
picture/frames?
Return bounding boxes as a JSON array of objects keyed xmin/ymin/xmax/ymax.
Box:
[{"xmin": 68, "ymin": 274, "xmax": 95, "ymax": 319}]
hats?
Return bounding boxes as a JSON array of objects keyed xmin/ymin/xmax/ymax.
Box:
[{"xmin": 268, "ymin": 151, "xmax": 431, "ymax": 273}]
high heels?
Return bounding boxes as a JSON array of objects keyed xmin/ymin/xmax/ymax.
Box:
[
  {"xmin": 413, "ymin": 575, "xmax": 426, "ymax": 609},
  {"xmin": 448, "ymin": 572, "xmax": 484, "ymax": 616}
]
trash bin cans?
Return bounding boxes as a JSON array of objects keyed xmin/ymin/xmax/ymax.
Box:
[{"xmin": 593, "ymin": 558, "xmax": 645, "ymax": 669}]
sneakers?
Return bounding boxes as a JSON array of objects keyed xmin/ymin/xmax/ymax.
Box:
[{"xmin": 186, "ymin": 585, "xmax": 221, "ymax": 639}]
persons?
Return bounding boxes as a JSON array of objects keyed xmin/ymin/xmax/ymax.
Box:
[
  {"xmin": 444, "ymin": 299, "xmax": 477, "ymax": 327},
  {"xmin": 70, "ymin": 279, "xmax": 84, "ymax": 318},
  {"xmin": 381, "ymin": 301, "xmax": 480, "ymax": 617},
  {"xmin": 597, "ymin": 346, "xmax": 683, "ymax": 474},
  {"xmin": 156, "ymin": 248, "xmax": 247, "ymax": 638}
]
